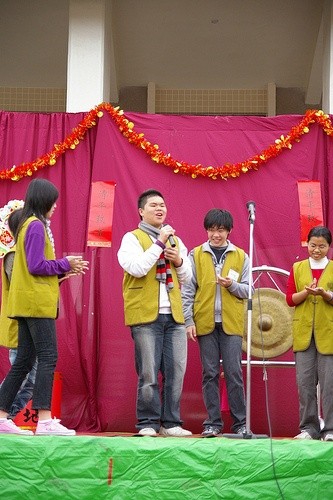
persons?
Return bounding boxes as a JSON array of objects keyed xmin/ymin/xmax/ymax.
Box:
[
  {"xmin": 117, "ymin": 190, "xmax": 192, "ymax": 437},
  {"xmin": 0, "ymin": 208, "xmax": 38, "ymax": 419},
  {"xmin": 182, "ymin": 209, "xmax": 255, "ymax": 437},
  {"xmin": 286, "ymin": 225, "xmax": 333, "ymax": 442},
  {"xmin": 0, "ymin": 179, "xmax": 89, "ymax": 436}
]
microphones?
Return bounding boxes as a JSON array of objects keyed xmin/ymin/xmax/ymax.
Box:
[
  {"xmin": 246, "ymin": 201, "xmax": 256, "ymax": 222},
  {"xmin": 162, "ymin": 223, "xmax": 176, "ymax": 248}
]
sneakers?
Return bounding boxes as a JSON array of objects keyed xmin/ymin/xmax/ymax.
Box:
[
  {"xmin": 294, "ymin": 430, "xmax": 312, "ymax": 439},
  {"xmin": 201, "ymin": 424, "xmax": 221, "ymax": 435},
  {"xmin": 139, "ymin": 427, "xmax": 156, "ymax": 436},
  {"xmin": 237, "ymin": 427, "xmax": 253, "ymax": 435},
  {"xmin": 159, "ymin": 426, "xmax": 192, "ymax": 437},
  {"xmin": 0, "ymin": 419, "xmax": 34, "ymax": 435},
  {"xmin": 323, "ymin": 434, "xmax": 333, "ymax": 441},
  {"xmin": 35, "ymin": 417, "xmax": 76, "ymax": 436}
]
